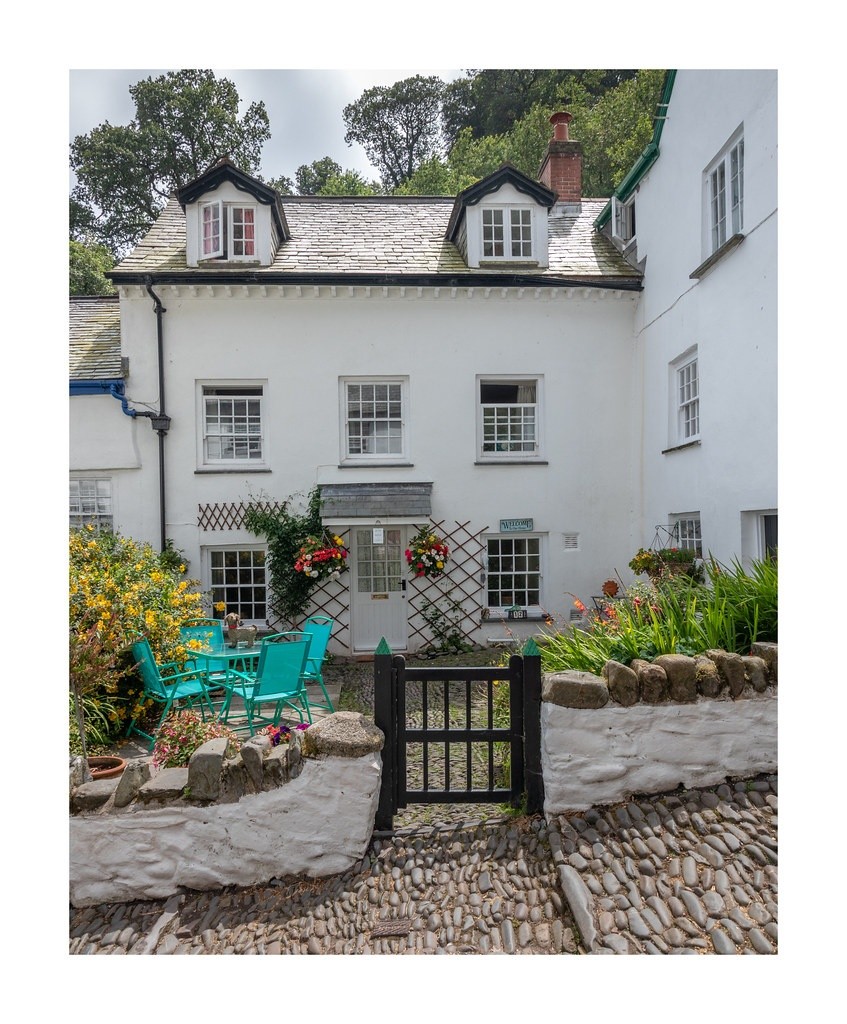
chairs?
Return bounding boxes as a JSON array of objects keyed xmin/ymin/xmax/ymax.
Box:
[{"xmin": 125, "ymin": 615, "xmax": 335, "ymax": 756}]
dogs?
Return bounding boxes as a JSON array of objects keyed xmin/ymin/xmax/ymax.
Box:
[{"xmin": 224, "ymin": 612, "xmax": 257, "ymax": 649}]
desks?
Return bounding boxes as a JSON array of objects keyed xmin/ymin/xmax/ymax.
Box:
[{"xmin": 184, "ymin": 637, "xmax": 274, "ymax": 722}]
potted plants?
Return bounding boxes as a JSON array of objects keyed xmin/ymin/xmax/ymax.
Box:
[{"xmin": 628, "ymin": 548, "xmax": 695, "ymax": 582}]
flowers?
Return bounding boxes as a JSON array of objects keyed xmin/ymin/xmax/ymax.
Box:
[
  {"xmin": 292, "ymin": 534, "xmax": 349, "ymax": 581},
  {"xmin": 570, "ymin": 580, "xmax": 662, "ymax": 628},
  {"xmin": 255, "ymin": 720, "xmax": 313, "ymax": 746},
  {"xmin": 404, "ymin": 526, "xmax": 453, "ymax": 579},
  {"xmin": 149, "ymin": 712, "xmax": 241, "ymax": 765}
]
